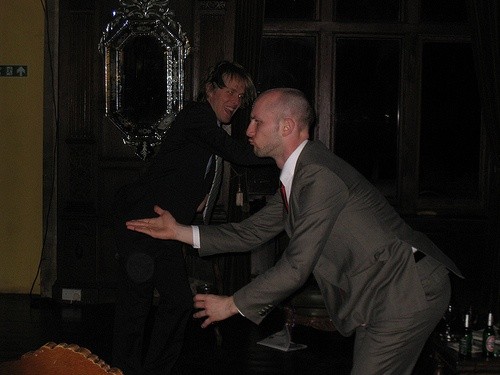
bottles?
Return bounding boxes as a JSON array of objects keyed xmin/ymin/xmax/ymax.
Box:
[
  {"xmin": 482, "ymin": 313, "xmax": 496, "ymax": 363},
  {"xmin": 458, "ymin": 311, "xmax": 472, "ymax": 361}
]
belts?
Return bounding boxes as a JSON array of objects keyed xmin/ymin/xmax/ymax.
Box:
[{"xmin": 414, "ymin": 250, "xmax": 426, "ymax": 263}]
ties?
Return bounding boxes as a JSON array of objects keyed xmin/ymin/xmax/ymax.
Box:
[
  {"xmin": 197, "ymin": 122, "xmax": 223, "ymax": 226},
  {"xmin": 279, "ymin": 183, "xmax": 289, "ymax": 215}
]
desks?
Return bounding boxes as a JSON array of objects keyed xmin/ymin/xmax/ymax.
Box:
[{"xmin": 426, "ymin": 330, "xmax": 500, "ymax": 375}]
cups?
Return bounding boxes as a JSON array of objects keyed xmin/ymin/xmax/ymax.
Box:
[
  {"xmin": 440, "ymin": 304, "xmax": 458, "ymax": 341},
  {"xmin": 196, "ymin": 283, "xmax": 216, "ymax": 323}
]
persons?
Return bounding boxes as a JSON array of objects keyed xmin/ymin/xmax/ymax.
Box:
[
  {"xmin": 125, "ymin": 88, "xmax": 464, "ymax": 375},
  {"xmin": 164, "ymin": 61, "xmax": 315, "ymax": 285}
]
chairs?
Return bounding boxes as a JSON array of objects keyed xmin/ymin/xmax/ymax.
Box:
[{"xmin": 0, "ymin": 342, "xmax": 124, "ymax": 375}]
